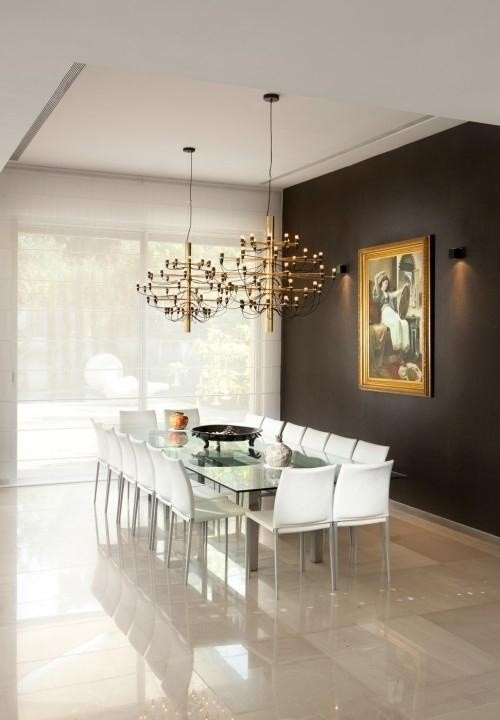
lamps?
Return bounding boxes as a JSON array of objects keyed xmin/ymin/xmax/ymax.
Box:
[{"xmin": 447, "ymin": 246, "xmax": 467, "ymax": 260}]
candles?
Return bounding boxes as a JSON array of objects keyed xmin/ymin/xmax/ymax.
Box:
[{"xmin": 355, "ymin": 233, "xmax": 437, "ymax": 400}]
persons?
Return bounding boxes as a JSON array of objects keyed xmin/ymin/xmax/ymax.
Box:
[{"xmin": 371, "ymin": 273, "xmax": 411, "ymax": 366}]
